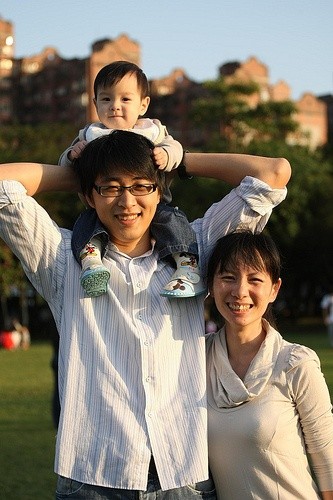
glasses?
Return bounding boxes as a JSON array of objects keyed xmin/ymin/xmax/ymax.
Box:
[{"xmin": 93, "ymin": 183, "xmax": 158, "ymax": 198}]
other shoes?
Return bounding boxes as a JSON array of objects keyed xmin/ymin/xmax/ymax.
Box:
[
  {"xmin": 80, "ymin": 264, "xmax": 111, "ymax": 298},
  {"xmin": 160, "ymin": 266, "xmax": 207, "ymax": 298}
]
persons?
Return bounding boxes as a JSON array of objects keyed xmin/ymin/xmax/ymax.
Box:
[
  {"xmin": 0, "ymin": 311, "xmax": 30, "ymax": 352},
  {"xmin": 189, "ymin": 225, "xmax": 332, "ymax": 500},
  {"xmin": 0, "ymin": 149, "xmax": 291, "ymax": 500},
  {"xmin": 57, "ymin": 61, "xmax": 207, "ymax": 296},
  {"xmin": 321, "ymin": 293, "xmax": 333, "ymax": 347},
  {"xmin": 202, "ymin": 307, "xmax": 216, "ymax": 335}
]
